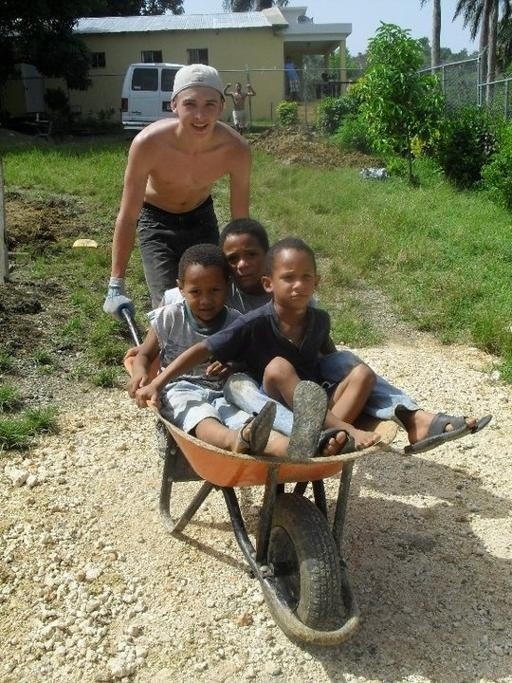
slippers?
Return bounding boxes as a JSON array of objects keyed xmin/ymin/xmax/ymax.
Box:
[
  {"xmin": 286, "ymin": 380, "xmax": 328, "ymax": 458},
  {"xmin": 404, "ymin": 412, "xmax": 493, "ymax": 454},
  {"xmin": 316, "ymin": 428, "xmax": 355, "ymax": 455},
  {"xmin": 239, "ymin": 400, "xmax": 276, "ymax": 457}
]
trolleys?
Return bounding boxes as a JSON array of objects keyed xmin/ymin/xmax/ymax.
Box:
[{"xmin": 116, "ymin": 271, "xmax": 391, "ymax": 649}]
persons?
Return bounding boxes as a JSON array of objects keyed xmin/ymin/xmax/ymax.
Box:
[
  {"xmin": 224, "ymin": 82, "xmax": 256, "ymax": 136},
  {"xmin": 124, "ymin": 217, "xmax": 379, "ymax": 464},
  {"xmin": 102, "ymin": 63, "xmax": 252, "ymax": 322},
  {"xmin": 284, "ymin": 56, "xmax": 300, "ymax": 102},
  {"xmin": 134, "ymin": 237, "xmax": 492, "ymax": 456}
]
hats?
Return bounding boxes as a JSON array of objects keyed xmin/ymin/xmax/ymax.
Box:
[{"xmin": 171, "ymin": 63, "xmax": 226, "ymax": 104}]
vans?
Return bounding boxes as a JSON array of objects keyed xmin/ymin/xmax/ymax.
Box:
[{"xmin": 121, "ymin": 61, "xmax": 189, "ymax": 142}]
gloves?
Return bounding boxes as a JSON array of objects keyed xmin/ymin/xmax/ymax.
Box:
[{"xmin": 103, "ymin": 277, "xmax": 136, "ymax": 323}]
